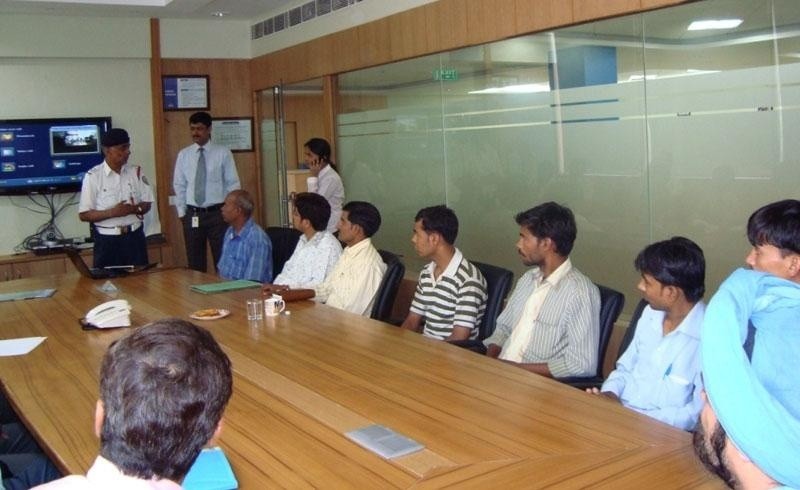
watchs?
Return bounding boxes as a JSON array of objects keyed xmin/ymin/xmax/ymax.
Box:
[{"xmin": 136, "ymin": 204, "xmax": 144, "ymax": 213}]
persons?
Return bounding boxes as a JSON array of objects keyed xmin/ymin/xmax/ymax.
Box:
[
  {"xmin": 25, "ymin": 316, "xmax": 233, "ymax": 489},
  {"xmin": 743, "ymin": 199, "xmax": 800, "ymax": 285},
  {"xmin": 269, "ymin": 200, "xmax": 388, "ymax": 319},
  {"xmin": 303, "ymin": 137, "xmax": 345, "ymax": 248},
  {"xmin": 399, "ymin": 203, "xmax": 489, "ymax": 342},
  {"xmin": 172, "ymin": 112, "xmax": 243, "ymax": 272},
  {"xmin": 260, "ymin": 191, "xmax": 344, "ymax": 306},
  {"xmin": 482, "ymin": 201, "xmax": 602, "ymax": 378},
  {"xmin": 691, "ymin": 267, "xmax": 800, "ymax": 489},
  {"xmin": 215, "ymin": 189, "xmax": 274, "ymax": 284},
  {"xmin": 585, "ymin": 236, "xmax": 707, "ymax": 431},
  {"xmin": 78, "ymin": 128, "xmax": 155, "ymax": 268},
  {"xmin": 1, "ymin": 420, "xmax": 66, "ymax": 489}
]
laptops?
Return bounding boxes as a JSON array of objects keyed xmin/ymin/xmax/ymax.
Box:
[{"xmin": 62, "ymin": 244, "xmax": 130, "ymax": 281}]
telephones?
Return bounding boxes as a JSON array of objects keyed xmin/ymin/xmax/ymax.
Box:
[{"xmin": 79, "ymin": 299, "xmax": 133, "ymax": 331}]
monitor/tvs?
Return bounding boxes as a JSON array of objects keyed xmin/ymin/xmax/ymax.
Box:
[{"xmin": 0, "ymin": 115, "xmax": 113, "ymax": 197}]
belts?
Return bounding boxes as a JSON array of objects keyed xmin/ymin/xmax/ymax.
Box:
[
  {"xmin": 187, "ymin": 205, "xmax": 219, "ymax": 212},
  {"xmin": 97, "ymin": 222, "xmax": 141, "ymax": 235}
]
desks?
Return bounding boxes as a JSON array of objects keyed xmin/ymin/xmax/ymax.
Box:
[{"xmin": 0, "ymin": 263, "xmax": 737, "ymax": 490}]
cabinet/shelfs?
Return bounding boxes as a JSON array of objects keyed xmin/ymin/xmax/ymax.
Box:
[{"xmin": 0, "ymin": 237, "xmax": 175, "ymax": 279}]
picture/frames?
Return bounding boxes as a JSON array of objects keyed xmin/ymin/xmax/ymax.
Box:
[
  {"xmin": 210, "ymin": 116, "xmax": 254, "ymax": 153},
  {"xmin": 161, "ymin": 73, "xmax": 211, "ymax": 113}
]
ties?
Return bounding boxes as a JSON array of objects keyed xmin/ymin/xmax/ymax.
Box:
[{"xmin": 195, "ymin": 149, "xmax": 206, "ymax": 204}]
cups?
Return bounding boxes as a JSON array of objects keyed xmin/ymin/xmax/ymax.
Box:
[
  {"xmin": 247, "ymin": 298, "xmax": 262, "ymax": 321},
  {"xmin": 264, "ymin": 298, "xmax": 285, "ymax": 317}
]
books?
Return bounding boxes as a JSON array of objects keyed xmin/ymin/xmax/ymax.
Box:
[{"xmin": 187, "ymin": 278, "xmax": 263, "ymax": 296}]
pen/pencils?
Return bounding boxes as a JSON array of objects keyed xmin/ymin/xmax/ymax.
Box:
[
  {"xmin": 10, "ymin": 297, "xmax": 35, "ymax": 300},
  {"xmin": 104, "ymin": 265, "xmax": 135, "ymax": 269}
]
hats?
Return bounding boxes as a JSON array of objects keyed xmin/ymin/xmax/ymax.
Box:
[{"xmin": 102, "ymin": 129, "xmax": 129, "ymax": 146}]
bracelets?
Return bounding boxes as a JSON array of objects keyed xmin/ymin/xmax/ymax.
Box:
[{"xmin": 283, "ymin": 285, "xmax": 289, "ymax": 292}]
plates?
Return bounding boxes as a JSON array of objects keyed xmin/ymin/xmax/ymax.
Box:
[{"xmin": 190, "ymin": 309, "xmax": 230, "ymax": 321}]
